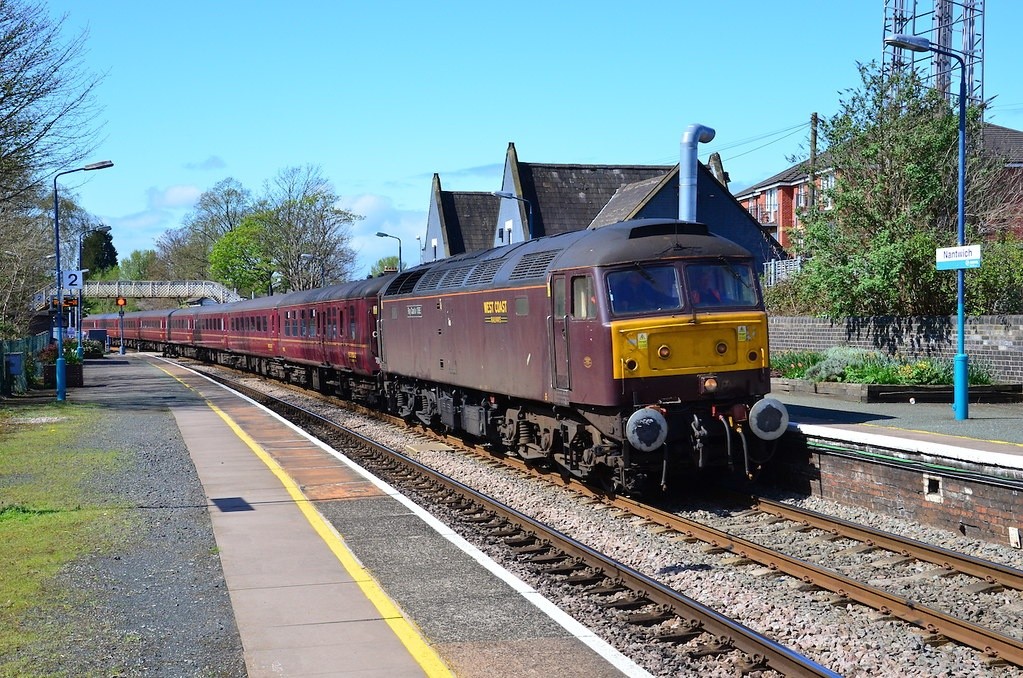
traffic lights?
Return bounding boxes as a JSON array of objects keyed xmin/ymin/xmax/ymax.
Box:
[{"xmin": 115, "ymin": 297, "xmax": 126, "ymax": 306}]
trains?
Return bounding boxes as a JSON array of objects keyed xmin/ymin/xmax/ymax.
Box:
[{"xmin": 79, "ymin": 215, "xmax": 792, "ymax": 503}]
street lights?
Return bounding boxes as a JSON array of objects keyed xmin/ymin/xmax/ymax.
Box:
[
  {"xmin": 76, "ymin": 225, "xmax": 112, "ymax": 364},
  {"xmin": 373, "ymin": 231, "xmax": 403, "ymax": 274},
  {"xmin": 489, "ymin": 191, "xmax": 535, "ymax": 242},
  {"xmin": 299, "ymin": 251, "xmax": 325, "ymax": 288},
  {"xmin": 885, "ymin": 34, "xmax": 972, "ymax": 420},
  {"xmin": 54, "ymin": 159, "xmax": 115, "ymax": 401}
]
